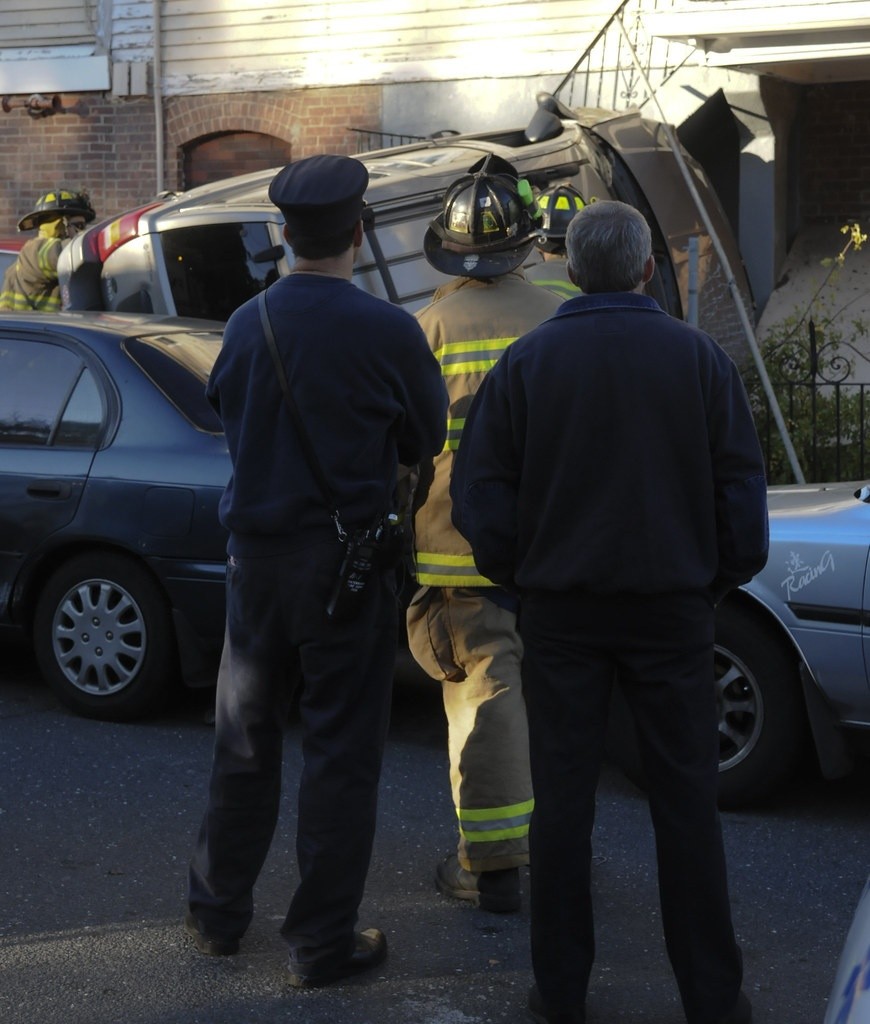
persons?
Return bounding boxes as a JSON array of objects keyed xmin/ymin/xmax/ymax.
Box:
[
  {"xmin": 405, "ymin": 157, "xmax": 592, "ymax": 920},
  {"xmin": 444, "ymin": 198, "xmax": 770, "ymax": 1023},
  {"xmin": 0, "ymin": 189, "xmax": 97, "ymax": 312},
  {"xmin": 181, "ymin": 154, "xmax": 452, "ymax": 983}
]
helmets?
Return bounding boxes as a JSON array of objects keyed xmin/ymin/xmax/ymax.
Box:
[
  {"xmin": 532, "ymin": 182, "xmax": 588, "ymax": 254},
  {"xmin": 17, "ymin": 191, "xmax": 97, "ymax": 230},
  {"xmin": 423, "ymin": 174, "xmax": 538, "ymax": 277}
]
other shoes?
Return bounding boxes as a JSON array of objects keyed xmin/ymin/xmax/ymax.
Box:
[
  {"xmin": 731, "ymin": 991, "xmax": 752, "ymax": 1024},
  {"xmin": 527, "ymin": 983, "xmax": 582, "ymax": 1023}
]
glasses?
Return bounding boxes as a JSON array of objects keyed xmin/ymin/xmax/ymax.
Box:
[{"xmin": 71, "ymin": 222, "xmax": 85, "ymax": 229}]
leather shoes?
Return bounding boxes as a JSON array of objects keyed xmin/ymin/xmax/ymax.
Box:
[
  {"xmin": 283, "ymin": 929, "xmax": 386, "ymax": 987},
  {"xmin": 436, "ymin": 857, "xmax": 522, "ymax": 912},
  {"xmin": 185, "ymin": 912, "xmax": 239, "ymax": 955}
]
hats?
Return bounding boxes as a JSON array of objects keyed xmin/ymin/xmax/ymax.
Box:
[{"xmin": 269, "ymin": 155, "xmax": 369, "ymax": 234}]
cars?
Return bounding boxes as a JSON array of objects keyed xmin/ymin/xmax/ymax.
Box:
[
  {"xmin": 0, "ymin": 308, "xmax": 245, "ymax": 722},
  {"xmin": 709, "ymin": 476, "xmax": 870, "ymax": 809}
]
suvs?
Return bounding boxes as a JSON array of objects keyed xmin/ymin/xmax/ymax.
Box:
[{"xmin": 58, "ymin": 93, "xmax": 756, "ymax": 401}]
